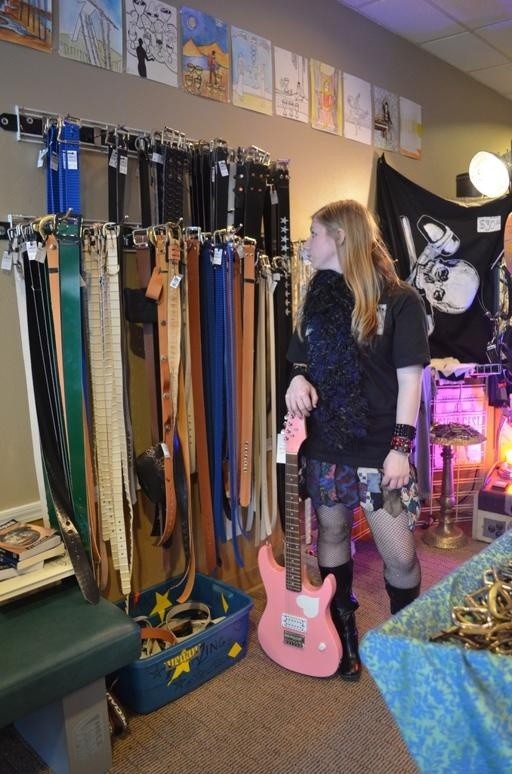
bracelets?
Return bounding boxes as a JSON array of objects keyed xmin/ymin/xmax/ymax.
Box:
[
  {"xmin": 286, "ymin": 364, "xmax": 311, "ymax": 387},
  {"xmin": 392, "ymin": 422, "xmax": 417, "ymax": 437},
  {"xmin": 389, "ymin": 435, "xmax": 414, "ymax": 453}
]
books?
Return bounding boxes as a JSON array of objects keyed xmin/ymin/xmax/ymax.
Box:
[{"xmin": 0, "ymin": 518, "xmax": 66, "ymax": 582}]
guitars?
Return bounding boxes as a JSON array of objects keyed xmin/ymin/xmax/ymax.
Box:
[{"xmin": 256, "ymin": 410, "xmax": 343, "ymax": 678}]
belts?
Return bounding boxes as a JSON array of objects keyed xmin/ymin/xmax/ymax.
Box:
[
  {"xmin": 5, "ymin": 113, "xmax": 292, "ymax": 602},
  {"xmin": 105, "ymin": 691, "xmax": 128, "ymax": 739},
  {"xmin": 133, "ymin": 602, "xmax": 211, "ymax": 647}
]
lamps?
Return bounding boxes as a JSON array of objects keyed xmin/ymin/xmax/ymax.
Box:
[
  {"xmin": 468, "ymin": 151, "xmax": 512, "ymax": 199},
  {"xmin": 422, "ymin": 423, "xmax": 488, "ymax": 551}
]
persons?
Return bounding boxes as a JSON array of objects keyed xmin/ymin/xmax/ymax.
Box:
[{"xmin": 283, "ymin": 198, "xmax": 432, "ymax": 683}]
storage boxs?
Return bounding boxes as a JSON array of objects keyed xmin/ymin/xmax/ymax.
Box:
[{"xmin": 113, "ymin": 569, "xmax": 253, "ymax": 714}]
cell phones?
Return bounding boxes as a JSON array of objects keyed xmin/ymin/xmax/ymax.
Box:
[{"xmin": 492, "ymin": 478, "xmax": 509, "ymax": 491}]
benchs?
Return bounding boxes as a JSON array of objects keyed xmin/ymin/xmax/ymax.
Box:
[{"xmin": 0, "ymin": 582, "xmax": 140, "ymax": 774}]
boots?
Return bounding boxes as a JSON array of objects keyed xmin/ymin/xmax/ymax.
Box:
[{"xmin": 331, "ymin": 595, "xmax": 362, "ymax": 680}]
